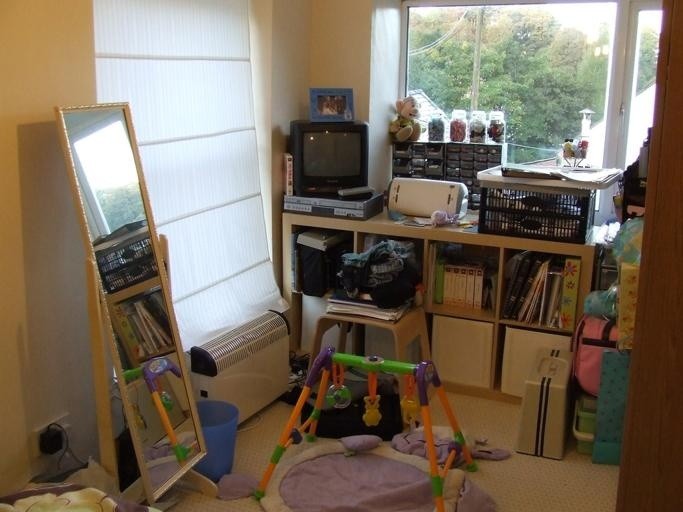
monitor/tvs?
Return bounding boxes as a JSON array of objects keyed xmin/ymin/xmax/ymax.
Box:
[{"xmin": 290, "ymin": 120, "xmax": 368, "ymax": 194}]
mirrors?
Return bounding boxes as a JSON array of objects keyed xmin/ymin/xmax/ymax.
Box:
[{"xmin": 55, "ymin": 101, "xmax": 207, "ymax": 507}]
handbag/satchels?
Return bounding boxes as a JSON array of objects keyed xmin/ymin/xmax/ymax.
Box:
[{"xmin": 572, "ymin": 314, "xmax": 619, "ymax": 398}]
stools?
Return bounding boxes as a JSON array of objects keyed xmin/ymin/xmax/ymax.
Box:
[{"xmin": 310, "ymin": 307, "xmax": 436, "ymax": 433}]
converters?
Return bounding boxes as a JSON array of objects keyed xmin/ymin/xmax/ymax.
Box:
[{"xmin": 39, "ymin": 429, "xmax": 63, "ymax": 455}]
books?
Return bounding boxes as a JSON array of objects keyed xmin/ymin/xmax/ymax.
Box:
[
  {"xmin": 436, "ymin": 257, "xmax": 572, "ymax": 327},
  {"xmin": 283, "ymin": 152, "xmax": 294, "ymax": 196},
  {"xmin": 113, "ymin": 290, "xmax": 174, "ymax": 361},
  {"xmin": 327, "ymin": 295, "xmax": 411, "ymax": 326}
]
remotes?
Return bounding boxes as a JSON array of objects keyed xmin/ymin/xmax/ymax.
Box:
[{"xmin": 337, "ymin": 185, "xmax": 373, "ymax": 196}]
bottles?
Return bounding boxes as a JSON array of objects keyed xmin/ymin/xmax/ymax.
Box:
[
  {"xmin": 449, "ymin": 110, "xmax": 467, "ymax": 142},
  {"xmin": 563, "ymin": 138, "xmax": 573, "ymax": 157},
  {"xmin": 469, "ymin": 111, "xmax": 486, "ymax": 144},
  {"xmin": 428, "ymin": 111, "xmax": 445, "ymax": 143},
  {"xmin": 487, "ymin": 111, "xmax": 506, "ymax": 144}
]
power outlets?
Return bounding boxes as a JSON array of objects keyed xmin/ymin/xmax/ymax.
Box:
[{"xmin": 30, "ymin": 413, "xmax": 69, "ymax": 459}]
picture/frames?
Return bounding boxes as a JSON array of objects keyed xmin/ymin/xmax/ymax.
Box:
[{"xmin": 309, "ymin": 88, "xmax": 354, "ymax": 122}]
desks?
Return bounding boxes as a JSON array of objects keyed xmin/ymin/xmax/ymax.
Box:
[
  {"xmin": 106, "ymin": 276, "xmax": 191, "ymax": 412},
  {"xmin": 281, "ymin": 203, "xmax": 601, "ymax": 405}
]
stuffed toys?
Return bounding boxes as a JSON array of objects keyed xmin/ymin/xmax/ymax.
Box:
[{"xmin": 390, "ymin": 96, "xmax": 423, "ymax": 142}]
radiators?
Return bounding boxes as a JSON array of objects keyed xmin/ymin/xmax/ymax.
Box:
[{"xmin": 184, "ymin": 309, "xmax": 290, "ymax": 423}]
